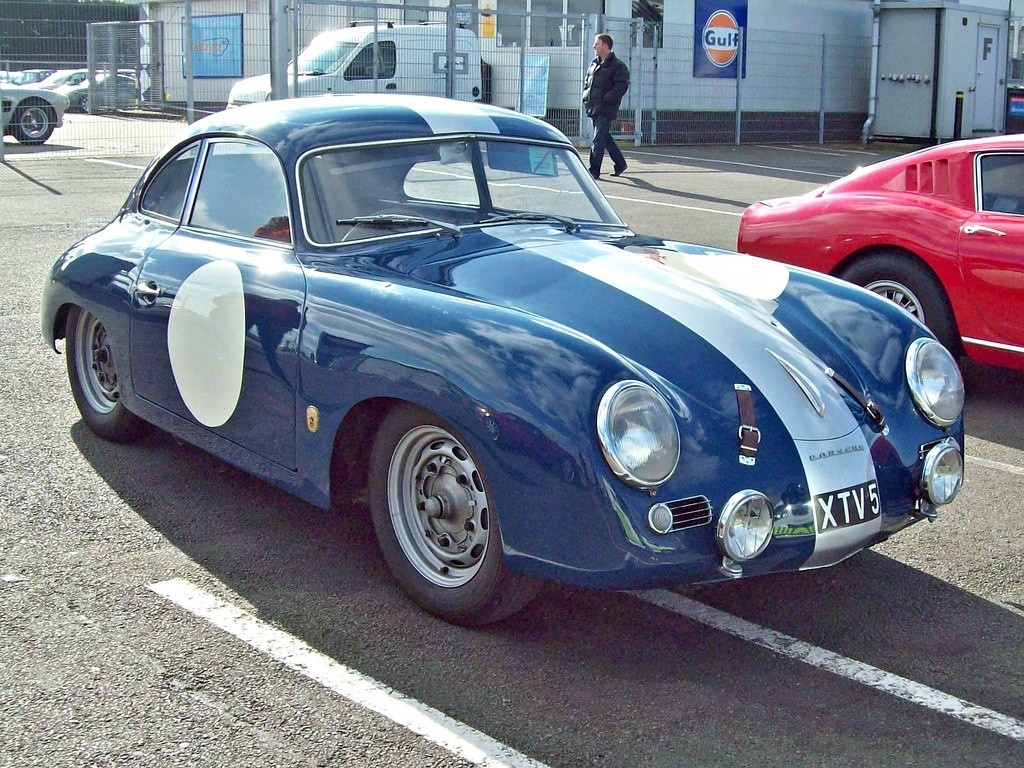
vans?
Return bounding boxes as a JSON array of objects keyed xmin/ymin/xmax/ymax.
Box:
[{"xmin": 226, "ymin": 23, "xmax": 482, "ymax": 108}]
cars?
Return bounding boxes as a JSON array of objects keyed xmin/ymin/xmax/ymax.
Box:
[
  {"xmin": 54, "ymin": 73, "xmax": 138, "ymax": 113},
  {"xmin": 0, "ymin": 83, "xmax": 65, "ymax": 145},
  {"xmin": 737, "ymin": 133, "xmax": 1024, "ymax": 375},
  {"xmin": 41, "ymin": 94, "xmax": 967, "ymax": 628},
  {"xmin": 0, "ymin": 70, "xmax": 101, "ymax": 90}
]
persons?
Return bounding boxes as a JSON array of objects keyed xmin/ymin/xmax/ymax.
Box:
[{"xmin": 582, "ymin": 33, "xmax": 630, "ymax": 180}]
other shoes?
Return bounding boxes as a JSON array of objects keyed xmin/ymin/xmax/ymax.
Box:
[
  {"xmin": 592, "ymin": 172, "xmax": 601, "ymax": 179},
  {"xmin": 609, "ymin": 164, "xmax": 627, "ymax": 176}
]
